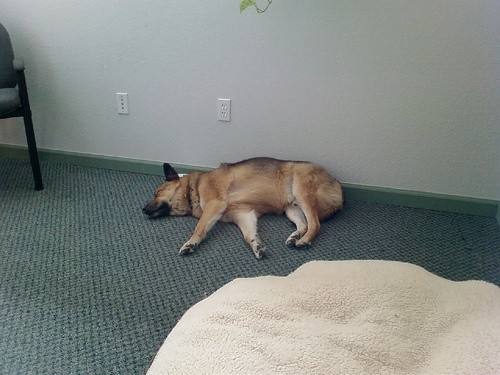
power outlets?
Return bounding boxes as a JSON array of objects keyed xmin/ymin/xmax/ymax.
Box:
[
  {"xmin": 217, "ymin": 98, "xmax": 231, "ymax": 122},
  {"xmin": 116, "ymin": 93, "xmax": 129, "ymax": 114}
]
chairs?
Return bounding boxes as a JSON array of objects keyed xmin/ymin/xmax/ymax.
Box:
[{"xmin": 1, "ymin": 23, "xmax": 43, "ymax": 191}]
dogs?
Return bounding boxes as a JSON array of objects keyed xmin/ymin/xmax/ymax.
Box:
[{"xmin": 141, "ymin": 156, "xmax": 344, "ymax": 260}]
